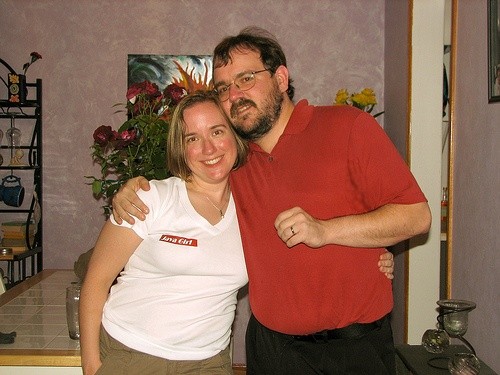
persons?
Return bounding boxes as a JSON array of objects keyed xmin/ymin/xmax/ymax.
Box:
[
  {"xmin": 111, "ymin": 27, "xmax": 432, "ymax": 375},
  {"xmin": 78, "ymin": 91, "xmax": 395, "ymax": 375}
]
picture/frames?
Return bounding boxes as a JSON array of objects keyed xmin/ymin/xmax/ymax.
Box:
[{"xmin": 487, "ymin": 0, "xmax": 500, "ymax": 104}]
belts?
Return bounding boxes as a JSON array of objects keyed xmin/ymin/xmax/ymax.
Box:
[{"xmin": 294, "ymin": 312, "xmax": 392, "ymax": 344}]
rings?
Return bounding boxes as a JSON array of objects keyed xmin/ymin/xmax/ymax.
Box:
[{"xmin": 291, "ymin": 227, "xmax": 294, "ymax": 233}]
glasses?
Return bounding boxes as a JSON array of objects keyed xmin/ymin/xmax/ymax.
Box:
[{"xmin": 210, "ymin": 69, "xmax": 268, "ymax": 102}]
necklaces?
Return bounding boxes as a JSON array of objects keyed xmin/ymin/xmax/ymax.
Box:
[{"xmin": 208, "ymin": 198, "xmax": 224, "ymax": 218}]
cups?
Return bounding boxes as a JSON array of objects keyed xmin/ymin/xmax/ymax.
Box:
[
  {"xmin": 66, "ymin": 286, "xmax": 81, "ymax": 339},
  {"xmin": 0, "ymin": 175, "xmax": 25, "ymax": 208}
]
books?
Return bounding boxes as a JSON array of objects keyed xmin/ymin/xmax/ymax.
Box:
[{"xmin": 0, "ymin": 221, "xmax": 36, "ymax": 254}]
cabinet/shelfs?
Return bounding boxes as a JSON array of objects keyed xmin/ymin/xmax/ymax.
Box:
[{"xmin": 0, "ymin": 58, "xmax": 45, "ymax": 282}]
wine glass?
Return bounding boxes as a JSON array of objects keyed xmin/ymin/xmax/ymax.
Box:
[{"xmin": 0, "ymin": 111, "xmax": 21, "ymax": 147}]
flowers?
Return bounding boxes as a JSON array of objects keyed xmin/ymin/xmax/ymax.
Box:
[
  {"xmin": 330, "ymin": 86, "xmax": 385, "ymax": 121},
  {"xmin": 82, "ymin": 78, "xmax": 190, "ymax": 223}
]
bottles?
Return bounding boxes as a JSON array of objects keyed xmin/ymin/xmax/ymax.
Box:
[
  {"xmin": 422, "ymin": 328, "xmax": 450, "ymax": 354},
  {"xmin": 447, "ymin": 352, "xmax": 481, "ymax": 375}
]
